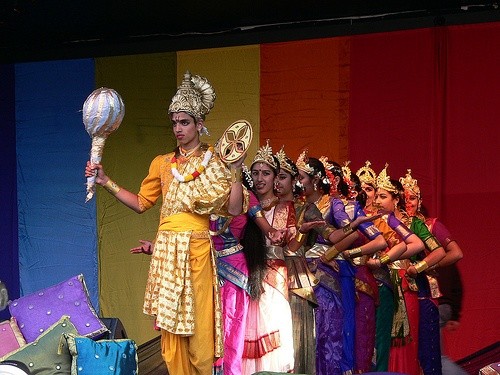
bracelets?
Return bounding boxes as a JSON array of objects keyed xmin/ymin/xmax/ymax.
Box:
[
  {"xmin": 103, "ymin": 179, "xmax": 120, "ymax": 195},
  {"xmin": 325, "ymin": 245, "xmax": 339, "ymax": 261},
  {"xmin": 414, "ymin": 260, "xmax": 429, "ymax": 273},
  {"xmin": 343, "ymin": 224, "xmax": 353, "ymax": 236},
  {"xmin": 379, "ymin": 254, "xmax": 391, "ymax": 266},
  {"xmin": 232, "ymin": 167, "xmax": 243, "ymax": 183},
  {"xmin": 295, "ymin": 230, "xmax": 308, "ymax": 242},
  {"xmin": 348, "ymin": 247, "xmax": 363, "ymax": 258}
]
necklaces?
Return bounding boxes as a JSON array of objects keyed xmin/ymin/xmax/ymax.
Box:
[{"xmin": 171, "ymin": 147, "xmax": 214, "ymax": 182}]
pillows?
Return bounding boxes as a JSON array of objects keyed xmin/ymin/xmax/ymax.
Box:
[{"xmin": 0, "ymin": 273, "xmax": 138, "ymax": 375}]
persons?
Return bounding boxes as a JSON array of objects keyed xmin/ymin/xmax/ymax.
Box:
[
  {"xmin": 84, "ymin": 71, "xmax": 248, "ymax": 375},
  {"xmin": 242, "ymin": 145, "xmax": 464, "ymax": 375},
  {"xmin": 130, "ymin": 164, "xmax": 287, "ymax": 375}
]
